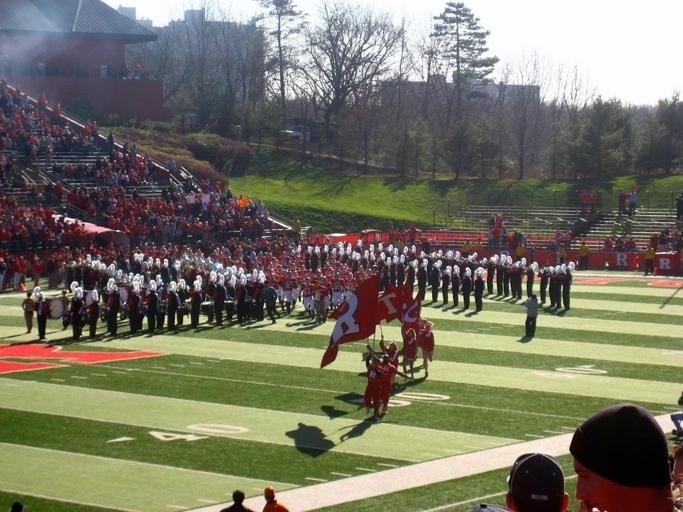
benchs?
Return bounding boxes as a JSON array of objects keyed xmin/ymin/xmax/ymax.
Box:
[
  {"xmin": 405, "ymin": 193, "xmax": 682, "ymax": 254},
  {"xmin": 0, "ymin": 99, "xmax": 245, "ymax": 264}
]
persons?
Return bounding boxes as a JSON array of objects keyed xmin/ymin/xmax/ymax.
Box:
[
  {"xmin": 11, "ymin": 501, "xmax": 23, "ymax": 512},
  {"xmin": 1, "ymin": 82, "xmax": 176, "ymax": 187},
  {"xmin": 401, "ymin": 318, "xmax": 435, "ymax": 383},
  {"xmin": 577, "ymin": 188, "xmax": 682, "ymax": 276},
  {"xmin": 220, "ymin": 486, "xmax": 290, "ymax": 512},
  {"xmin": 0, "ymin": 174, "xmax": 351, "ymax": 341},
  {"xmin": 506, "ymin": 403, "xmax": 683, "ymax": 512},
  {"xmin": 365, "ymin": 340, "xmax": 412, "ymax": 417},
  {"xmin": 343, "ymin": 213, "xmax": 575, "ymax": 338}
]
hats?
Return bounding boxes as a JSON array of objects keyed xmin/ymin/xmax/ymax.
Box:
[
  {"xmin": 570, "ymin": 403, "xmax": 669, "ymax": 487},
  {"xmin": 507, "ymin": 453, "xmax": 564, "ymax": 501}
]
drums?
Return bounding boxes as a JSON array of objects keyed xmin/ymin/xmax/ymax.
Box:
[
  {"xmin": 225, "ymin": 301, "xmax": 234, "ymax": 313},
  {"xmin": 99, "ymin": 306, "xmax": 110, "ymax": 321},
  {"xmin": 120, "ymin": 304, "xmax": 130, "ymax": 320},
  {"xmin": 48, "ymin": 298, "xmax": 66, "ymax": 319},
  {"xmin": 178, "ymin": 305, "xmax": 188, "ymax": 315},
  {"xmin": 160, "ymin": 304, "xmax": 168, "ymax": 315},
  {"xmin": 202, "ymin": 302, "xmax": 212, "ymax": 314}
]
